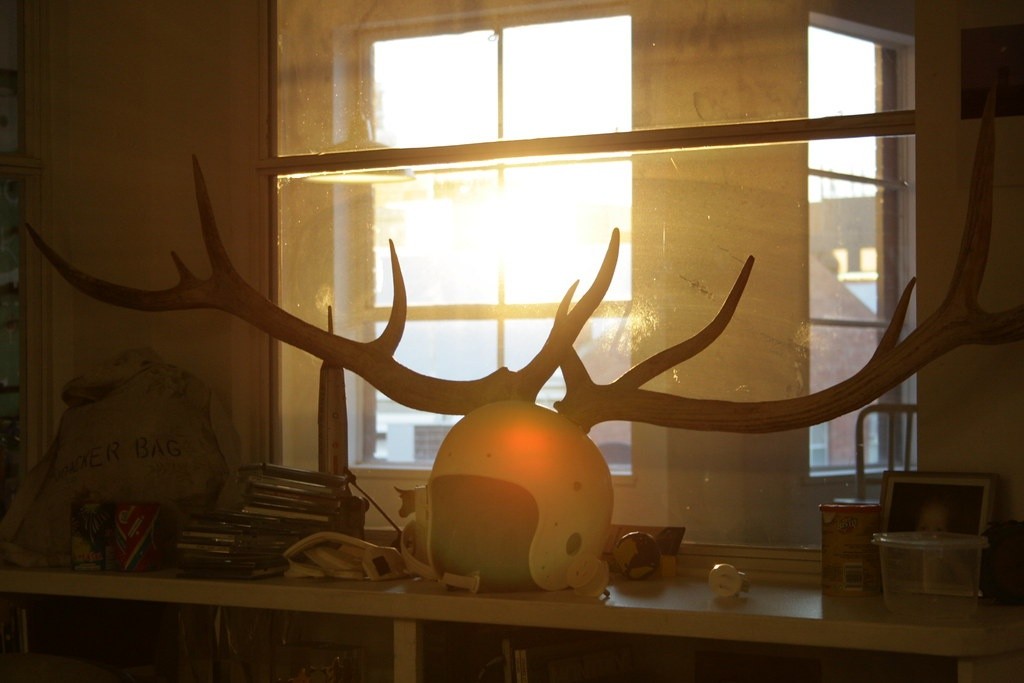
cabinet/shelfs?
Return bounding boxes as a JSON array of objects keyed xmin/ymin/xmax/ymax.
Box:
[{"xmin": 0, "ymin": 569, "xmax": 1024, "ymax": 683}]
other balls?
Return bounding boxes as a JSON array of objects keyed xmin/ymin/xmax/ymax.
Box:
[{"xmin": 612, "ymin": 531, "xmax": 660, "ymax": 581}]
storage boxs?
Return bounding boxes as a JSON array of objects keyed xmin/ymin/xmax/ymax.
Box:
[
  {"xmin": 871, "ymin": 530, "xmax": 990, "ymax": 600},
  {"xmin": 821, "ymin": 504, "xmax": 879, "ymax": 601}
]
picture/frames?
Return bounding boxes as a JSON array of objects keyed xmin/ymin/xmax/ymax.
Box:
[{"xmin": 879, "ymin": 469, "xmax": 1002, "ymax": 599}]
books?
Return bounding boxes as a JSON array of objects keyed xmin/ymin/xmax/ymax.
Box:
[{"xmin": 172, "ymin": 457, "xmax": 352, "ymax": 575}]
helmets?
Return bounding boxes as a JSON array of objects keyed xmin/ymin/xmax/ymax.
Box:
[{"xmin": 427, "ymin": 401, "xmax": 614, "ymax": 591}]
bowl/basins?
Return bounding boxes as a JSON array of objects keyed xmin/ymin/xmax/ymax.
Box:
[{"xmin": 870, "ymin": 530, "xmax": 991, "ymax": 619}]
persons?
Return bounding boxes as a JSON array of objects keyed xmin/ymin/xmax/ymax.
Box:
[{"xmin": 913, "ymin": 503, "xmax": 948, "ymax": 532}]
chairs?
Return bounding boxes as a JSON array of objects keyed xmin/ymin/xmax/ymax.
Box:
[{"xmin": 857, "ymin": 403, "xmax": 918, "ymax": 500}]
